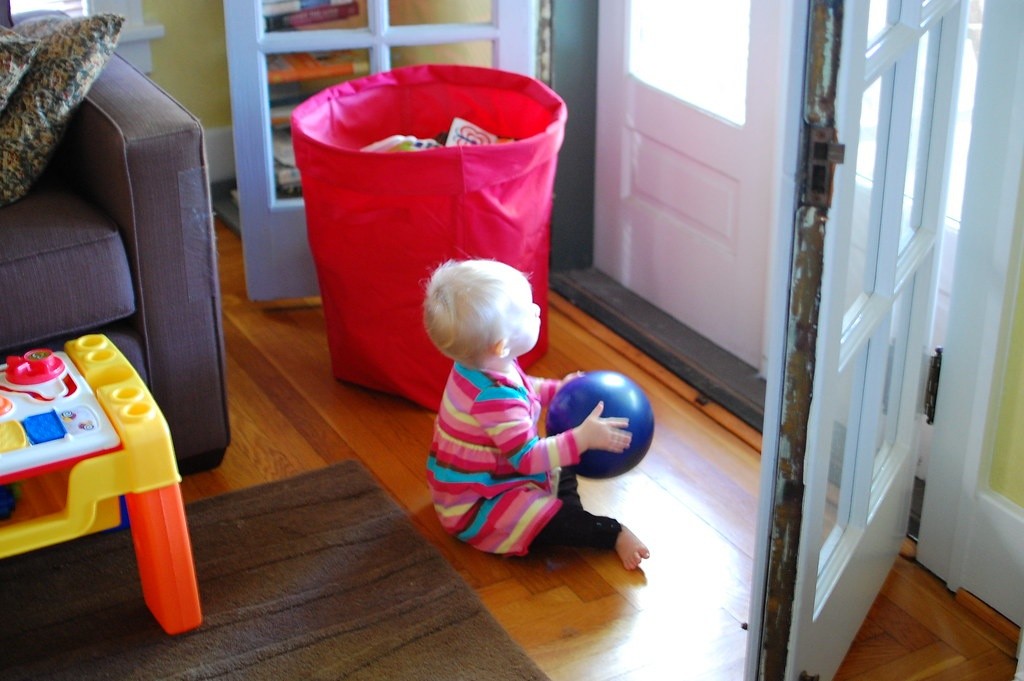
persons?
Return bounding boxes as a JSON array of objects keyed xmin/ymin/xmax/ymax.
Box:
[{"xmin": 423, "ymin": 259, "xmax": 651, "ymax": 570}]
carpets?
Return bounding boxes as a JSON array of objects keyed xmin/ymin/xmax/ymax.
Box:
[{"xmin": 0, "ymin": 462, "xmax": 553, "ymax": 681}]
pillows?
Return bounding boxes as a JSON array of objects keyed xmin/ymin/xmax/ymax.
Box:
[{"xmin": 0, "ymin": 12, "xmax": 127, "ymax": 204}]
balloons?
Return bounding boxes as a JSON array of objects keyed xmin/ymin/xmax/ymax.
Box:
[{"xmin": 545, "ymin": 370, "xmax": 656, "ymax": 479}]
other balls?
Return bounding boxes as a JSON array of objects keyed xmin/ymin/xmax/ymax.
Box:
[{"xmin": 544, "ymin": 369, "xmax": 655, "ymax": 480}]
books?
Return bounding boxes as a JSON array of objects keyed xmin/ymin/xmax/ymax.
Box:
[{"xmin": 262, "ymin": 0, "xmax": 372, "ymax": 189}]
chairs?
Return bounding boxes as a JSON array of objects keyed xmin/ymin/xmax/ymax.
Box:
[{"xmin": 0, "ymin": 0, "xmax": 231, "ymax": 476}]
